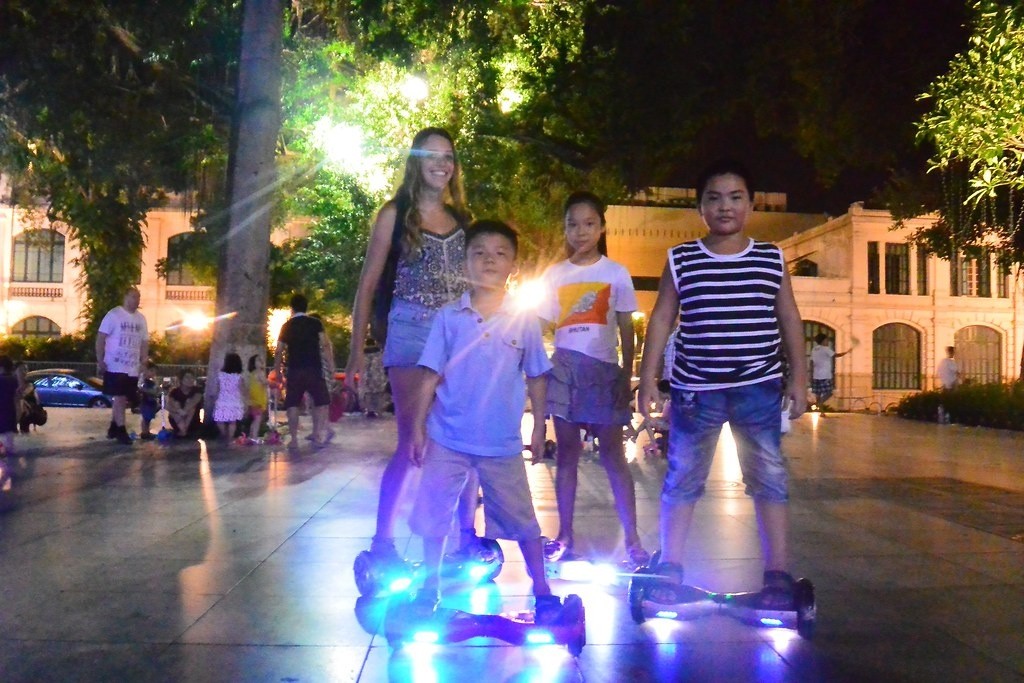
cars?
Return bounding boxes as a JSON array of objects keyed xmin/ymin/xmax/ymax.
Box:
[{"xmin": 25, "ymin": 367, "xmax": 116, "ymax": 410}]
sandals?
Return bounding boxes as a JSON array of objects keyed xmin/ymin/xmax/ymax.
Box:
[
  {"xmin": 646, "ymin": 561, "xmax": 684, "ymax": 604},
  {"xmin": 371, "ymin": 536, "xmax": 407, "ymax": 570},
  {"xmin": 756, "ymin": 569, "xmax": 795, "ymax": 611},
  {"xmin": 535, "ymin": 594, "xmax": 562, "ymax": 624},
  {"xmin": 411, "ymin": 588, "xmax": 441, "ymax": 618},
  {"xmin": 457, "ymin": 529, "xmax": 497, "ymax": 566}
]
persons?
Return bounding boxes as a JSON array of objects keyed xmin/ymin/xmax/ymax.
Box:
[
  {"xmin": 346, "ymin": 128, "xmax": 493, "ymax": 569},
  {"xmin": 407, "ymin": 220, "xmax": 564, "ymax": 625},
  {"xmin": 934, "ymin": 345, "xmax": 970, "ymax": 389},
  {"xmin": 0, "ymin": 355, "xmax": 40, "ymax": 433},
  {"xmin": 97, "ymin": 287, "xmax": 393, "ymax": 451},
  {"xmin": 533, "ymin": 191, "xmax": 649, "ymax": 571},
  {"xmin": 619, "ymin": 323, "xmax": 677, "ymax": 457},
  {"xmin": 809, "ymin": 333, "xmax": 852, "ymax": 415},
  {"xmin": 637, "ymin": 165, "xmax": 811, "ymax": 610}
]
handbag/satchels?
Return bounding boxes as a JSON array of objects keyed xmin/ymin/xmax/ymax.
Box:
[{"xmin": 31, "ymin": 405, "xmax": 47, "ymax": 426}]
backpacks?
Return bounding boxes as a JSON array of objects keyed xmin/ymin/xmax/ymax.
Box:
[{"xmin": 371, "ymin": 200, "xmax": 465, "ymax": 344}]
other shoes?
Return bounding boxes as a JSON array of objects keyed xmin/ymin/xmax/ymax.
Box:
[
  {"xmin": 141, "ymin": 431, "xmax": 156, "ymax": 440},
  {"xmin": 287, "ymin": 441, "xmax": 297, "ymax": 450},
  {"xmin": 625, "ymin": 542, "xmax": 649, "ymax": 566},
  {"xmin": 546, "ymin": 529, "xmax": 574, "ymax": 563},
  {"xmin": 313, "ymin": 431, "xmax": 332, "ymax": 447},
  {"xmin": 643, "ymin": 443, "xmax": 658, "ymax": 450},
  {"xmin": 107, "ymin": 421, "xmax": 133, "ymax": 445},
  {"xmin": 820, "ymin": 414, "xmax": 825, "ymax": 417}
]
